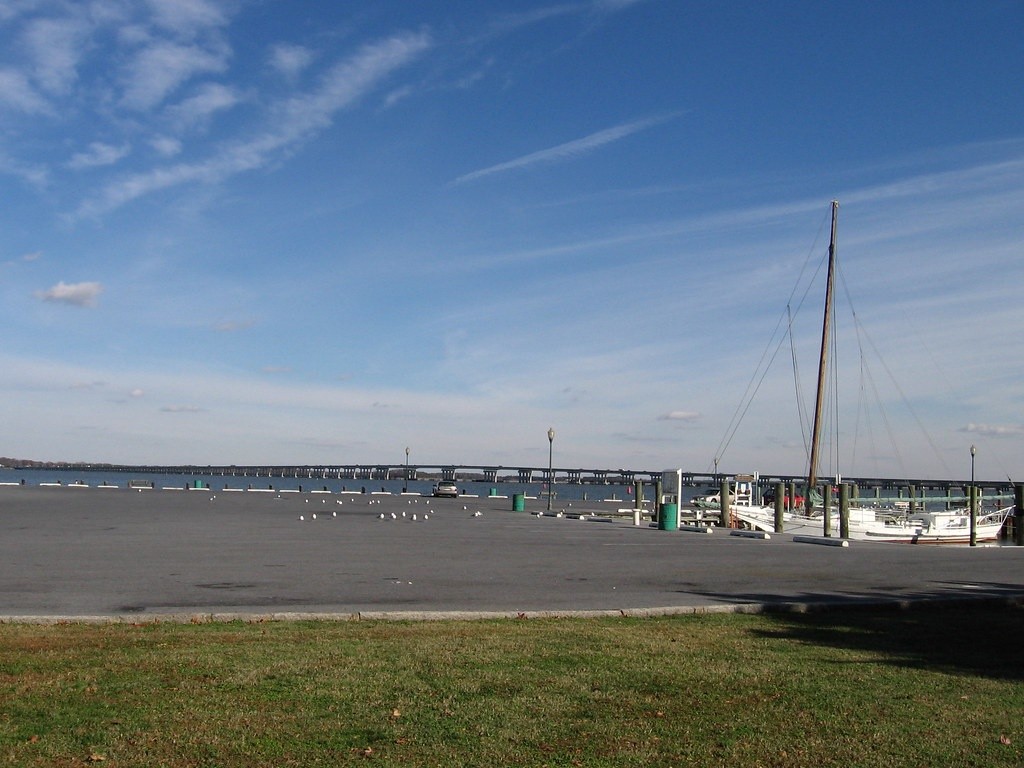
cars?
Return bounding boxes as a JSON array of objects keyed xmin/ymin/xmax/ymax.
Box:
[
  {"xmin": 433, "ymin": 480, "xmax": 458, "ymax": 498},
  {"xmin": 760, "ymin": 486, "xmax": 806, "ymax": 511},
  {"xmin": 690, "ymin": 487, "xmax": 736, "ymax": 510}
]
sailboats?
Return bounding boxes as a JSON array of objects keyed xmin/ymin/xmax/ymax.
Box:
[{"xmin": 688, "ymin": 198, "xmax": 1019, "ymax": 546}]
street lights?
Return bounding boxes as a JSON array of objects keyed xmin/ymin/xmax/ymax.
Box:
[
  {"xmin": 970, "ymin": 444, "xmax": 976, "ymax": 487},
  {"xmin": 403, "ymin": 446, "xmax": 410, "ymax": 493},
  {"xmin": 547, "ymin": 427, "xmax": 555, "ymax": 510}
]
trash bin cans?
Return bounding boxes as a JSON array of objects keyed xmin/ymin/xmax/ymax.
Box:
[
  {"xmin": 513, "ymin": 493, "xmax": 525, "ymax": 512},
  {"xmin": 658, "ymin": 503, "xmax": 677, "ymax": 530},
  {"xmin": 490, "ymin": 488, "xmax": 496, "ymax": 496}
]
